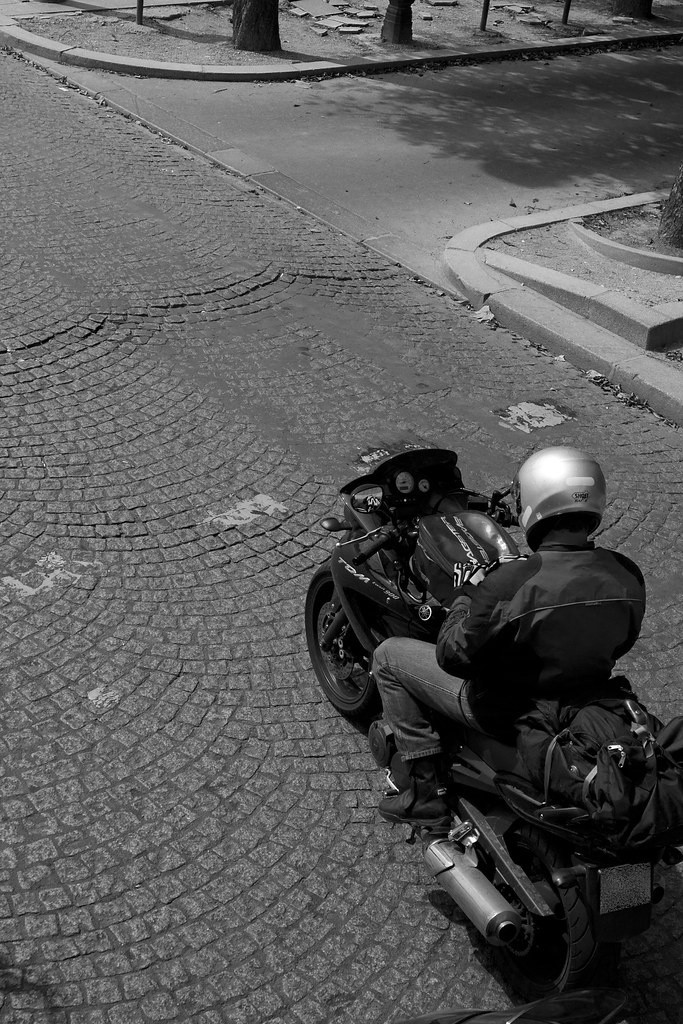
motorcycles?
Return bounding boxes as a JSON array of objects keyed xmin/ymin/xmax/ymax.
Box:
[{"xmin": 303, "ymin": 431, "xmax": 683, "ymax": 1011}]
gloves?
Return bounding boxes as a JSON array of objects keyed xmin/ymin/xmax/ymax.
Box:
[{"xmin": 450, "ymin": 560, "xmax": 498, "ymax": 602}]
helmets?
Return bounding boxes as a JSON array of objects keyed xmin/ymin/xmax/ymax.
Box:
[{"xmin": 510, "ymin": 446, "xmax": 606, "ymax": 551}]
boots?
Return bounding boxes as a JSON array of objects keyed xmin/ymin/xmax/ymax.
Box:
[{"xmin": 377, "ymin": 753, "xmax": 453, "ymax": 827}]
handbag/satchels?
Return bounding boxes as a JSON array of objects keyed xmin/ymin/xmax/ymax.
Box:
[{"xmin": 514, "ymin": 675, "xmax": 683, "ymax": 852}]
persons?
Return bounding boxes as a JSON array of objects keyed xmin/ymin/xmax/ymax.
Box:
[{"xmin": 367, "ymin": 444, "xmax": 650, "ymax": 829}]
